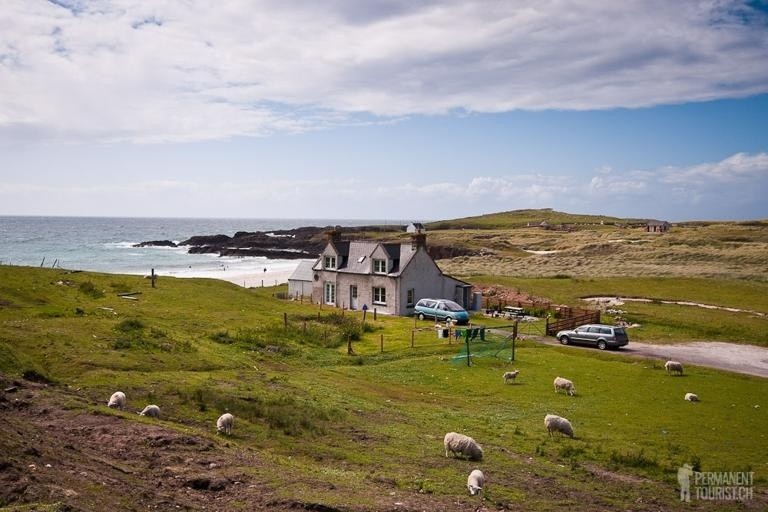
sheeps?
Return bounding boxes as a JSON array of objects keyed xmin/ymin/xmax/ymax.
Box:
[
  {"xmin": 544, "ymin": 414, "xmax": 573, "ymax": 439},
  {"xmin": 444, "ymin": 431, "xmax": 484, "ymax": 461},
  {"xmin": 106, "ymin": 391, "xmax": 127, "ymax": 411},
  {"xmin": 467, "ymin": 469, "xmax": 485, "ymax": 496},
  {"xmin": 664, "ymin": 361, "xmax": 684, "ymax": 376},
  {"xmin": 215, "ymin": 413, "xmax": 234, "ymax": 435},
  {"xmin": 684, "ymin": 392, "xmax": 698, "ymax": 401},
  {"xmin": 502, "ymin": 369, "xmax": 520, "ymax": 384},
  {"xmin": 137, "ymin": 404, "xmax": 161, "ymax": 419},
  {"xmin": 554, "ymin": 376, "xmax": 575, "ymax": 396}
]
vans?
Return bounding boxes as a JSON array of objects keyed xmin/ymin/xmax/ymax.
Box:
[{"xmin": 414, "ymin": 297, "xmax": 469, "ymax": 326}]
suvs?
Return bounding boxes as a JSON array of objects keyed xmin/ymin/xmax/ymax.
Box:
[{"xmin": 555, "ymin": 323, "xmax": 628, "ymax": 350}]
